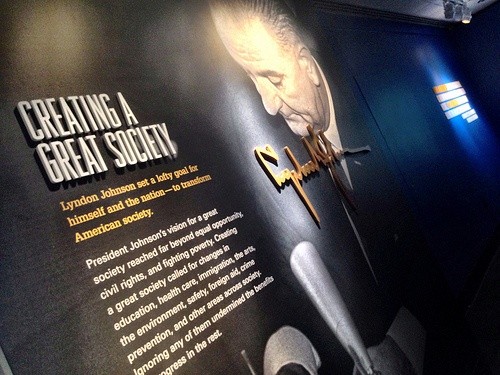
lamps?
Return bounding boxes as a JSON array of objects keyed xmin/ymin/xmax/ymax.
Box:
[
  {"xmin": 452, "ymin": 3, "xmax": 463, "ymax": 21},
  {"xmin": 461, "ymin": 5, "xmax": 474, "ymax": 24},
  {"xmin": 441, "ymin": 0, "xmax": 454, "ymax": 20}
]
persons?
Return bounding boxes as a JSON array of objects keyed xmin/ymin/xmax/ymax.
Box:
[{"xmin": 208, "ymin": 0, "xmax": 429, "ymax": 375}]
